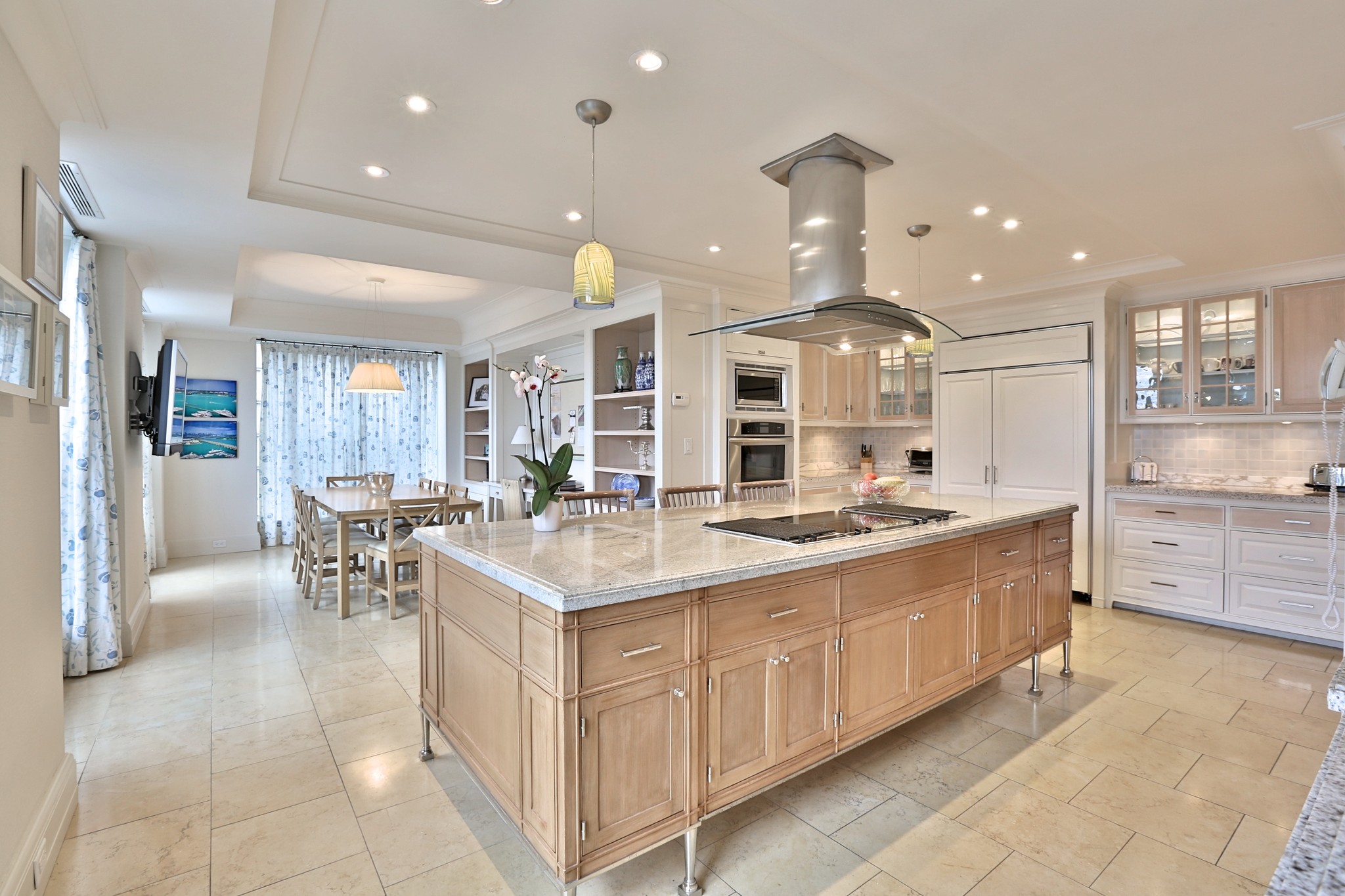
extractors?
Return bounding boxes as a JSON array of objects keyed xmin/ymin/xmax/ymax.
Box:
[{"xmin": 688, "ymin": 133, "xmax": 930, "ymax": 357}]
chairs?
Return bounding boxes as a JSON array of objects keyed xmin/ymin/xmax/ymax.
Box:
[
  {"xmin": 289, "ymin": 473, "xmax": 526, "ymax": 620},
  {"xmin": 563, "ymin": 477, "xmax": 795, "ymax": 518}
]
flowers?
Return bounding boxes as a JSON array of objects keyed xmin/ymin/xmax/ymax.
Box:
[{"xmin": 491, "ymin": 354, "xmax": 573, "ymax": 517}]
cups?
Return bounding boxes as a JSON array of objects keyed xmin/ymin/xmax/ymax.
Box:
[
  {"xmin": 880, "ymin": 346, "xmax": 933, "ymax": 370},
  {"xmin": 1136, "ymin": 363, "xmax": 1150, "ymax": 375},
  {"xmin": 1136, "ymin": 402, "xmax": 1178, "ymax": 409},
  {"xmin": 1172, "ymin": 361, "xmax": 1183, "ymax": 373},
  {"xmin": 1201, "ymin": 354, "xmax": 1255, "ymax": 372},
  {"xmin": 883, "ymin": 378, "xmax": 932, "ymax": 392},
  {"xmin": 882, "ymin": 404, "xmax": 932, "ymax": 416}
]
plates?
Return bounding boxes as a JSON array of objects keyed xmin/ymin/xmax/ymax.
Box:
[
  {"xmin": 611, "ymin": 473, "xmax": 640, "ymax": 501},
  {"xmin": 634, "ymin": 496, "xmax": 655, "ymax": 507}
]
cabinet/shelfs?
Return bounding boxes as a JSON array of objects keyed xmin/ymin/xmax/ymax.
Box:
[
  {"xmin": 592, "ymin": 311, "xmax": 662, "ymax": 515},
  {"xmin": 1270, "ymin": 277, "xmax": 1345, "ymax": 413},
  {"xmin": 1227, "ymin": 507, "xmax": 1344, "ymax": 642},
  {"xmin": 1106, "ymin": 492, "xmax": 1226, "ymax": 616},
  {"xmin": 419, "ymin": 506, "xmax": 1079, "ymax": 883},
  {"xmin": 799, "ymin": 341, "xmax": 933, "ymax": 425},
  {"xmin": 1125, "ymin": 289, "xmax": 1266, "ymax": 417},
  {"xmin": 463, "ymin": 358, "xmax": 492, "ymax": 486}
]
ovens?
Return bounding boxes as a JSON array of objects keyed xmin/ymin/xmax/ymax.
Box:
[{"xmin": 726, "ymin": 417, "xmax": 794, "ymax": 503}]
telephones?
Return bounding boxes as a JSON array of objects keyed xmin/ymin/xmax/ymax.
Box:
[{"xmin": 1318, "ymin": 338, "xmax": 1345, "ymax": 403}]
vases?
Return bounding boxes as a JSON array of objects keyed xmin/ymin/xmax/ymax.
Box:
[{"xmin": 530, "ymin": 495, "xmax": 564, "ymax": 532}]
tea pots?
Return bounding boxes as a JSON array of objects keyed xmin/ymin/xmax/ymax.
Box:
[{"xmin": 1150, "ymin": 357, "xmax": 1174, "ymax": 375}]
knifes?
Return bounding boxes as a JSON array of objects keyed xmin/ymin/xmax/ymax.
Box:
[{"xmin": 861, "ymin": 443, "xmax": 872, "ymax": 458}]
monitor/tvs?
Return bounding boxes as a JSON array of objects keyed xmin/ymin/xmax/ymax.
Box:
[{"xmin": 152, "ymin": 340, "xmax": 188, "ymax": 457}]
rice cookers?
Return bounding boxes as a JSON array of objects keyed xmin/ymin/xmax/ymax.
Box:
[{"xmin": 905, "ymin": 447, "xmax": 933, "ymax": 473}]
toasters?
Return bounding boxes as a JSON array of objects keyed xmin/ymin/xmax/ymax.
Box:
[{"xmin": 1304, "ymin": 463, "xmax": 1345, "ymax": 493}]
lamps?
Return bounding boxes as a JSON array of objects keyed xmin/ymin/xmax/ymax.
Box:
[
  {"xmin": 572, "ymin": 99, "xmax": 616, "ymax": 310},
  {"xmin": 344, "ymin": 276, "xmax": 406, "ymax": 393},
  {"xmin": 906, "ymin": 223, "xmax": 933, "ymax": 358}
]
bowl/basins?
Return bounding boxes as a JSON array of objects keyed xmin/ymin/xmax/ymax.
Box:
[{"xmin": 851, "ymin": 480, "xmax": 910, "ymax": 504}]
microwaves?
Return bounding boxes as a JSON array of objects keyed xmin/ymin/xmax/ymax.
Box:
[{"xmin": 726, "ymin": 359, "xmax": 794, "ymax": 418}]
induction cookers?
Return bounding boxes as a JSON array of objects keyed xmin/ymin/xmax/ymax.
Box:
[{"xmin": 700, "ymin": 505, "xmax": 971, "ymax": 547}]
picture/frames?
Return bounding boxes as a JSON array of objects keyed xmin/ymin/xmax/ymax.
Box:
[
  {"xmin": 467, "ymin": 375, "xmax": 488, "ymax": 408},
  {"xmin": 21, "ymin": 165, "xmax": 64, "ymax": 304},
  {"xmin": 30, "ymin": 305, "xmax": 70, "ymax": 408},
  {"xmin": 0, "ymin": 262, "xmax": 43, "ymax": 401}
]
